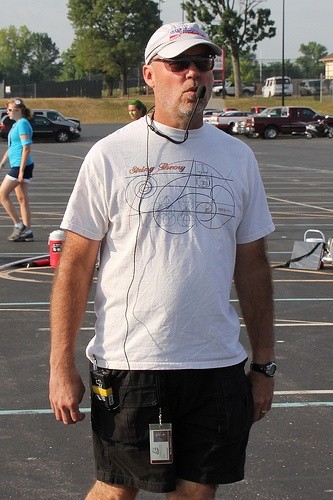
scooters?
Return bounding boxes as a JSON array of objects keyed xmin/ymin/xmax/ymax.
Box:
[{"xmin": 304, "ymin": 114, "xmax": 333, "ymax": 140}]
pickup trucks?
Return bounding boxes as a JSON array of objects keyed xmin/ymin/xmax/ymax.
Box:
[
  {"xmin": 212, "ymin": 81, "xmax": 255, "ymax": 97},
  {"xmin": 203, "ymin": 105, "xmax": 288, "ymax": 138},
  {"xmin": 245, "ymin": 106, "xmax": 333, "ymax": 140}
]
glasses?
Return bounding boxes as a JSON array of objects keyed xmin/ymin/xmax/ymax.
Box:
[
  {"xmin": 7, "ymin": 108, "xmax": 19, "ymax": 112},
  {"xmin": 151, "ymin": 53, "xmax": 220, "ymax": 72}
]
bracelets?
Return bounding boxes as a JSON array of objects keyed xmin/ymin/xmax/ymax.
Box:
[{"xmin": 0, "ymin": 162, "xmax": 4, "ymax": 168}]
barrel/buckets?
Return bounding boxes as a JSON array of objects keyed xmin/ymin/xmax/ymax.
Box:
[{"xmin": 48, "ymin": 230, "xmax": 65, "ymax": 266}]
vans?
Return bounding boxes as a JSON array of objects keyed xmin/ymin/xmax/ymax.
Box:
[
  {"xmin": 261, "ymin": 75, "xmax": 294, "ymax": 98},
  {"xmin": 299, "ymin": 79, "xmax": 328, "ymax": 96}
]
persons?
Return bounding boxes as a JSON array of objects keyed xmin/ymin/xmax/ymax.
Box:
[
  {"xmin": 0, "ymin": 97, "xmax": 34, "ymax": 242},
  {"xmin": 128, "ymin": 99, "xmax": 147, "ymax": 120},
  {"xmin": 48, "ymin": 22, "xmax": 278, "ymax": 500}
]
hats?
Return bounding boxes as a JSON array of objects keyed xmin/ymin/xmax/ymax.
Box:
[{"xmin": 145, "ymin": 22, "xmax": 222, "ymax": 65}]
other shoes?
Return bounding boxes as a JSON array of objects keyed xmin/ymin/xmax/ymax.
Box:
[
  {"xmin": 13, "ymin": 231, "xmax": 33, "ymax": 242},
  {"xmin": 7, "ymin": 225, "xmax": 26, "ymax": 240}
]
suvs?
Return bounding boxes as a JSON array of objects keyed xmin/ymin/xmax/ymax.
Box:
[
  {"xmin": 0, "ymin": 114, "xmax": 80, "ymax": 142},
  {"xmin": 0, "ymin": 109, "xmax": 80, "ymax": 134}
]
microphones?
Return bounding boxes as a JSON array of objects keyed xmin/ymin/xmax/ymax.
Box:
[{"xmin": 184, "ymin": 84, "xmax": 206, "ymax": 139}]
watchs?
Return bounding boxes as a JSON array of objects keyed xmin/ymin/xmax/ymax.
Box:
[{"xmin": 250, "ymin": 360, "xmax": 277, "ymax": 377}]
